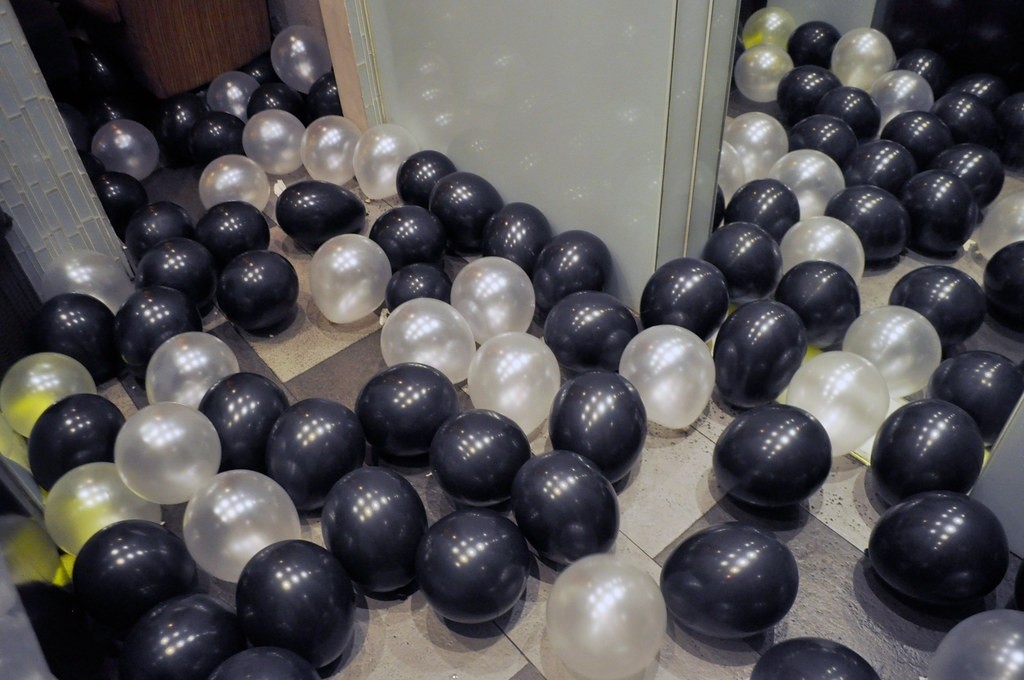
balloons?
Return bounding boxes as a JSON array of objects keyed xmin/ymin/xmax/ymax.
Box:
[
  {"xmin": 417, "ymin": 508, "xmax": 535, "ymax": 625},
  {"xmin": 0, "ymin": 0, "xmax": 1024, "ymax": 680}
]
